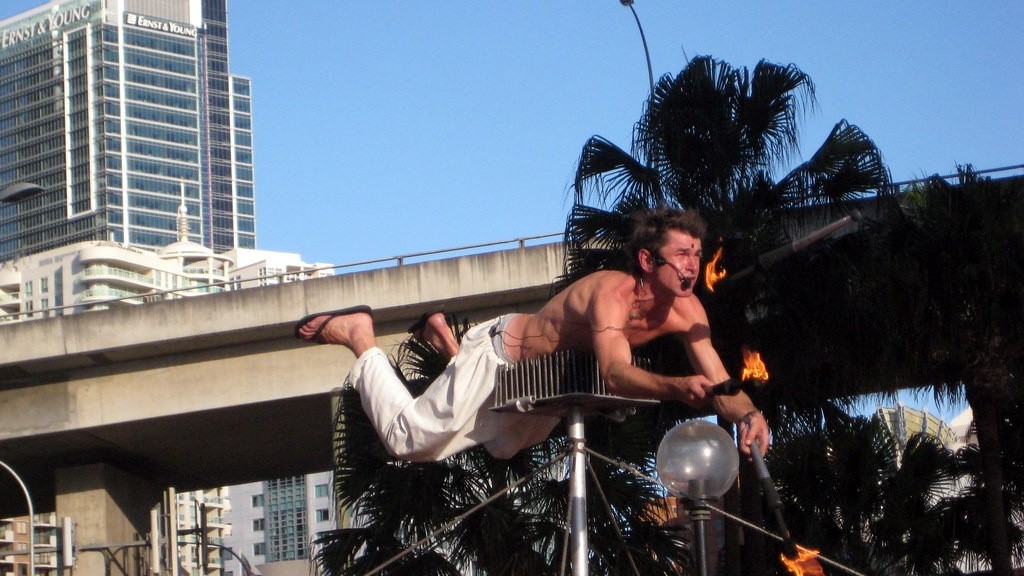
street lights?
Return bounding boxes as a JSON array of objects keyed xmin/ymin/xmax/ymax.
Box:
[
  {"xmin": 657, "ymin": 419, "xmax": 739, "ymax": 576},
  {"xmin": 618, "ymin": 0, "xmax": 661, "ymax": 213}
]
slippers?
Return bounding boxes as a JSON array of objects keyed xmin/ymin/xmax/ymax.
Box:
[
  {"xmin": 295, "ymin": 305, "xmax": 373, "ymax": 344},
  {"xmin": 408, "ymin": 304, "xmax": 444, "ymax": 342}
]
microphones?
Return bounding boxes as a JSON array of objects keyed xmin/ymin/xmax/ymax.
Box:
[
  {"xmin": 701, "ymin": 378, "xmax": 741, "ymax": 397},
  {"xmin": 652, "ymin": 256, "xmax": 690, "ymax": 289}
]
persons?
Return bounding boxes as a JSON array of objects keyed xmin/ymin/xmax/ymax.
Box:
[{"xmin": 295, "ymin": 204, "xmax": 769, "ymax": 465}]
